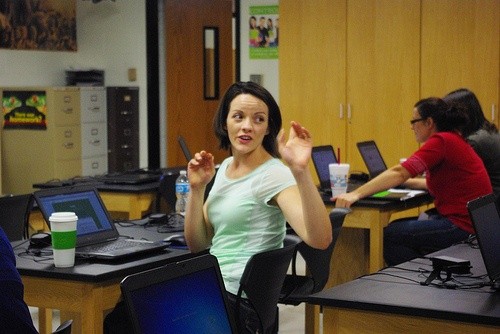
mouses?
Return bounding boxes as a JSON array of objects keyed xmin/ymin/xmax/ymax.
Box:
[{"xmin": 162, "ymin": 235, "xmax": 186, "ymax": 247}]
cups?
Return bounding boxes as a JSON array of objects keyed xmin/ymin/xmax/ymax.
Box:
[
  {"xmin": 49, "ymin": 211, "xmax": 78, "ymax": 268},
  {"xmin": 328, "ymin": 163, "xmax": 350, "ymax": 198}
]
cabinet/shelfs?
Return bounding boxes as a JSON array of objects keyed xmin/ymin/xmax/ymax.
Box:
[
  {"xmin": 278, "ymin": 0, "xmax": 421, "ymax": 185},
  {"xmin": 105, "ymin": 86, "xmax": 140, "ymax": 172},
  {"xmin": 0, "ymin": 87, "xmax": 108, "ymax": 195},
  {"xmin": 421, "ymin": 0, "xmax": 500, "ymax": 132}
]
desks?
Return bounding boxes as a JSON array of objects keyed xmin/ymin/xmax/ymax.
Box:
[
  {"xmin": 308, "ymin": 240, "xmax": 500, "ymax": 334},
  {"xmin": 27, "ymin": 166, "xmax": 187, "ymax": 234},
  {"xmin": 319, "ymin": 180, "xmax": 436, "ymax": 292},
  {"xmin": 11, "ymin": 215, "xmax": 213, "ymax": 334}
]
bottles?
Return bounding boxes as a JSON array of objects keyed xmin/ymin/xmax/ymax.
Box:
[{"xmin": 175, "ymin": 170, "xmax": 190, "ymax": 215}]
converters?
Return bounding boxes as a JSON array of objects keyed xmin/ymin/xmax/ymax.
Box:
[
  {"xmin": 31, "ymin": 232, "xmax": 51, "ymax": 249},
  {"xmin": 432, "ymin": 256, "xmax": 471, "ymax": 274},
  {"xmin": 149, "ymin": 214, "xmax": 168, "ymax": 224}
]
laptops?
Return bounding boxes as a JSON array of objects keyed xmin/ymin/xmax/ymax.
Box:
[
  {"xmin": 105, "ymin": 174, "xmax": 161, "ymax": 185},
  {"xmin": 468, "ymin": 193, "xmax": 499, "ymax": 290},
  {"xmin": 311, "ymin": 140, "xmax": 406, "ymax": 207},
  {"xmin": 33, "ymin": 185, "xmax": 171, "ymax": 265},
  {"xmin": 178, "ymin": 137, "xmax": 192, "ymax": 163}
]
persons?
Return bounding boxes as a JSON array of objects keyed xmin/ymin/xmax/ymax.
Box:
[
  {"xmin": 0, "ymin": 0, "xmax": 77, "ymax": 52},
  {"xmin": 249, "ymin": 15, "xmax": 279, "ymax": 47},
  {"xmin": 0, "ymin": 225, "xmax": 41, "ymax": 334},
  {"xmin": 330, "ymin": 96, "xmax": 494, "ymax": 268},
  {"xmin": 102, "ymin": 81, "xmax": 333, "ymax": 334},
  {"xmin": 391, "ymin": 87, "xmax": 500, "ymax": 213}
]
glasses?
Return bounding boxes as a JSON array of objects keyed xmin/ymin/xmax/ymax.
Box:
[{"xmin": 411, "ymin": 118, "xmax": 424, "ymax": 124}]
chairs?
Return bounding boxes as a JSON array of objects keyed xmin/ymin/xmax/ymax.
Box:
[
  {"xmin": 154, "ymin": 173, "xmax": 181, "ymax": 212},
  {"xmin": 233, "ymin": 207, "xmax": 352, "ymax": 334}
]
